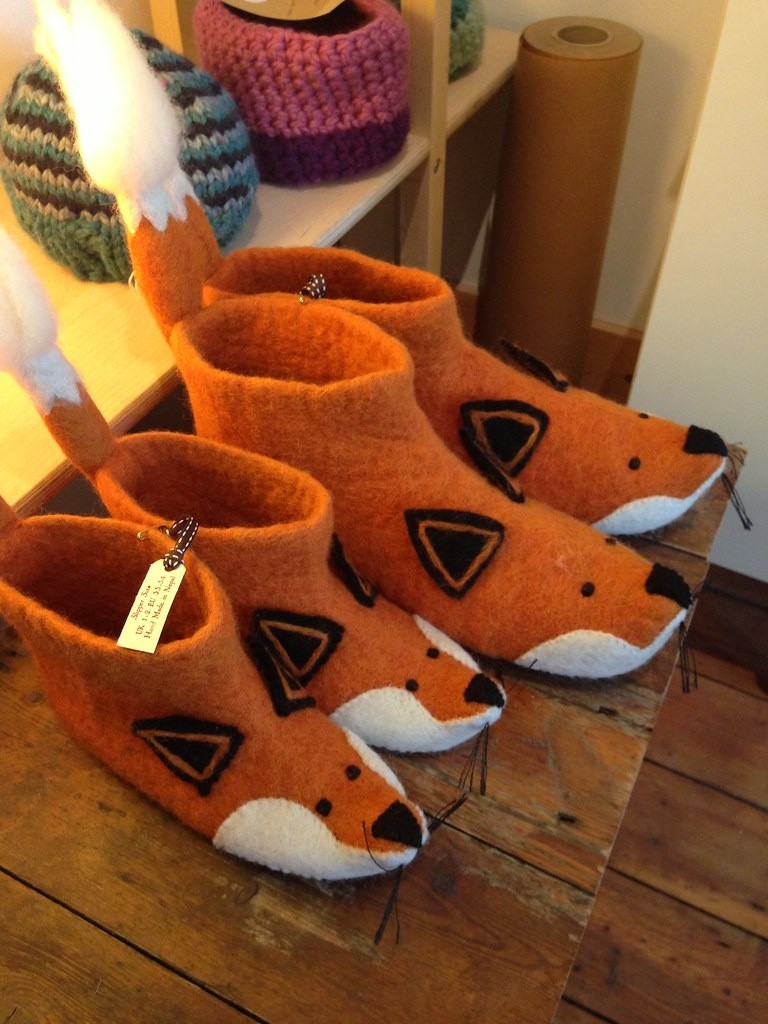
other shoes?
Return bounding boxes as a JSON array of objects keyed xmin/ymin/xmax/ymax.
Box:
[
  {"xmin": 1, "ymin": 230, "xmax": 509, "ymax": 754},
  {"xmin": 73, "ymin": 4, "xmax": 730, "ymax": 534},
  {"xmin": 29, "ymin": 1, "xmax": 694, "ymax": 677},
  {"xmin": 0, "ymin": 495, "xmax": 430, "ymax": 880}
]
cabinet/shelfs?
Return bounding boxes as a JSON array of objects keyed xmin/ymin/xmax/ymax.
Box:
[{"xmin": 0, "ymin": 0, "xmax": 751, "ymax": 1021}]
